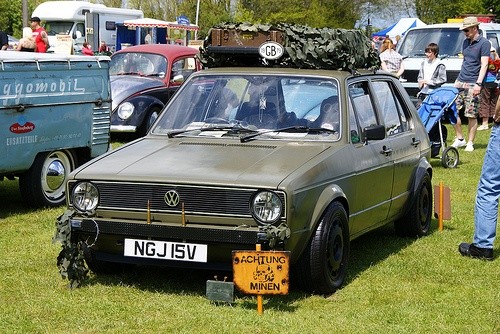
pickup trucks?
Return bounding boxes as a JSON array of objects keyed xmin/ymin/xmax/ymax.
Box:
[{"xmin": 0, "ymin": 53, "xmax": 112, "ymax": 206}]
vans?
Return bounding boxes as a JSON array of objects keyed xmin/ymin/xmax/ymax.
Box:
[{"xmin": 388, "ymin": 22, "xmax": 500, "ymax": 105}]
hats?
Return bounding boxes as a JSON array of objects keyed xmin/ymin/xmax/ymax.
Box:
[
  {"xmin": 29, "ymin": 17, "xmax": 40, "ymax": 21},
  {"xmin": 459, "ymin": 16, "xmax": 480, "ymax": 30}
]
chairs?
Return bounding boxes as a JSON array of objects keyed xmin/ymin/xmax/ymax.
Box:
[
  {"xmin": 312, "ymin": 96, "xmax": 339, "ymax": 131},
  {"xmin": 236, "ymin": 102, "xmax": 278, "ymax": 128}
]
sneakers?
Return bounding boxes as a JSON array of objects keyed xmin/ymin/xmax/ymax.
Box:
[
  {"xmin": 477, "ymin": 125, "xmax": 488, "ymax": 130},
  {"xmin": 452, "ymin": 137, "xmax": 466, "ymax": 148},
  {"xmin": 458, "ymin": 242, "xmax": 494, "ymax": 261},
  {"xmin": 465, "ymin": 142, "xmax": 474, "ymax": 151}
]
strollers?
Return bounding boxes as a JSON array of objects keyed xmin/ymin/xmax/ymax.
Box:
[{"xmin": 416, "ymin": 85, "xmax": 465, "ymax": 169}]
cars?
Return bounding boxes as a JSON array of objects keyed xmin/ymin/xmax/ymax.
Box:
[
  {"xmin": 107, "ymin": 43, "xmax": 203, "ymax": 140},
  {"xmin": 64, "ymin": 67, "xmax": 434, "ymax": 296}
]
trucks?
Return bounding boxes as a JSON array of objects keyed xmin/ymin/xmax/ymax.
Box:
[{"xmin": 31, "ymin": 1, "xmax": 144, "ymax": 58}]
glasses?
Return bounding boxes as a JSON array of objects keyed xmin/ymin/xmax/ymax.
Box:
[{"xmin": 462, "ymin": 29, "xmax": 469, "ymax": 32}]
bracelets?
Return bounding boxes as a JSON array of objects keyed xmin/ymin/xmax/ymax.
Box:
[{"xmin": 476, "ymin": 82, "xmax": 482, "ymax": 86}]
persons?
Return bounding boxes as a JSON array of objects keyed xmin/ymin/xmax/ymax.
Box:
[
  {"xmin": 416, "ymin": 43, "xmax": 446, "ymax": 109},
  {"xmin": 0, "ymin": 30, "xmax": 9, "ymax": 50},
  {"xmin": 380, "ymin": 39, "xmax": 405, "ymax": 79},
  {"xmin": 459, "ymin": 94, "xmax": 500, "ymax": 260},
  {"xmin": 145, "ymin": 31, "xmax": 152, "ymax": 44},
  {"xmin": 82, "ymin": 42, "xmax": 94, "ymax": 56},
  {"xmin": 447, "ymin": 17, "xmax": 491, "ymax": 151},
  {"xmin": 99, "ymin": 41, "xmax": 111, "ymax": 56},
  {"xmin": 18, "ymin": 28, "xmax": 37, "ymax": 52},
  {"xmin": 30, "ymin": 18, "xmax": 49, "ymax": 53},
  {"xmin": 372, "ymin": 35, "xmax": 400, "ymax": 49},
  {"xmin": 477, "ymin": 49, "xmax": 500, "ymax": 130}
]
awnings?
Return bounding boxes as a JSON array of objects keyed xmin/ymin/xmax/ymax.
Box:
[{"xmin": 116, "ymin": 19, "xmax": 199, "ymax": 45}]
